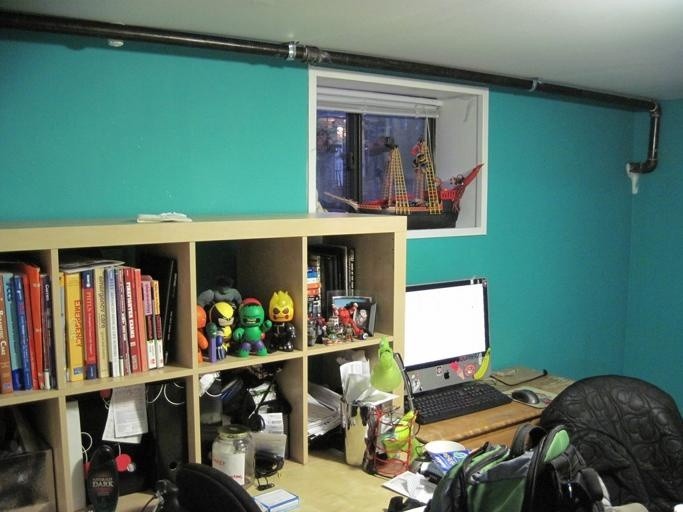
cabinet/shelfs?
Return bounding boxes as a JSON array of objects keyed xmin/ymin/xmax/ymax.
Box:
[{"xmin": 0, "ymin": 213, "xmax": 407, "ymax": 511}]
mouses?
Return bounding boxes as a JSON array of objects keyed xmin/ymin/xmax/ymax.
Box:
[{"xmin": 512, "ymin": 388, "xmax": 539, "ymax": 404}]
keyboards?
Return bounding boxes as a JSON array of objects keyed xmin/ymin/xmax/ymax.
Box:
[{"xmin": 404, "ymin": 383, "xmax": 510, "ymax": 424}]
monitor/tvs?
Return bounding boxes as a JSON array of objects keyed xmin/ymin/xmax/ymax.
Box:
[{"xmin": 405, "ymin": 276, "xmax": 490, "ymax": 394}]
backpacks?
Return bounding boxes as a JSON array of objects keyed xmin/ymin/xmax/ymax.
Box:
[{"xmin": 425, "ymin": 425, "xmax": 604, "ymax": 512}]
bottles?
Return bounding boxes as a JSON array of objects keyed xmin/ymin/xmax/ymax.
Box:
[
  {"xmin": 371, "ymin": 337, "xmax": 404, "ymax": 432},
  {"xmin": 212, "ymin": 425, "xmax": 256, "ymax": 489},
  {"xmin": 86, "ymin": 446, "xmax": 119, "ymax": 512}
]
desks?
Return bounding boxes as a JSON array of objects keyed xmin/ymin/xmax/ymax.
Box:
[{"xmin": 410, "ymin": 365, "xmax": 574, "ymax": 453}]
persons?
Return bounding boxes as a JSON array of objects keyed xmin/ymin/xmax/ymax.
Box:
[{"xmin": 194, "ymin": 275, "xmax": 296, "ymax": 365}]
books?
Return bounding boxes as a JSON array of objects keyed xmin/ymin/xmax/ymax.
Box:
[{"xmin": 0, "ymin": 258, "xmax": 165, "ymax": 392}]
